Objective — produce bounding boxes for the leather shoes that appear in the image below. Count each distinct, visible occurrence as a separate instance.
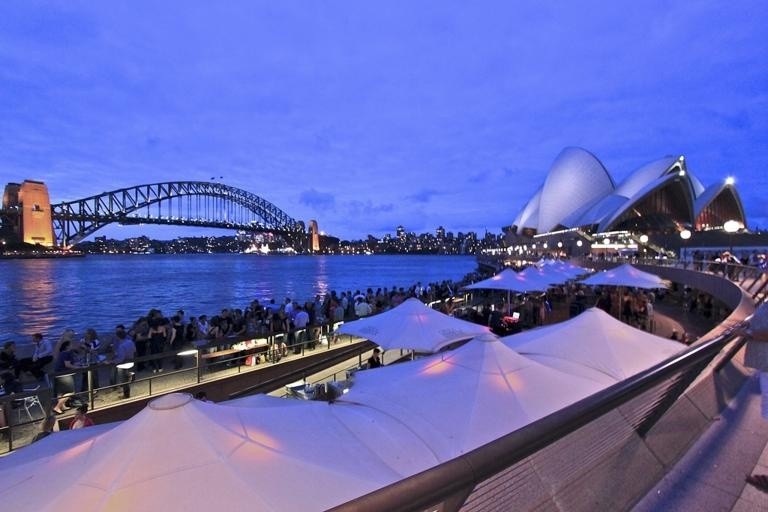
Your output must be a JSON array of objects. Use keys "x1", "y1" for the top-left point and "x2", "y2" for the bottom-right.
[{"x1": 53, "y1": 405, "x2": 71, "y2": 414}]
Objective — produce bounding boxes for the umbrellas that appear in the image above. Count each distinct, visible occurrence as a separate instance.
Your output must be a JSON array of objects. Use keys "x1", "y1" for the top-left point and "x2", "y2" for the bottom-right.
[{"x1": 0, "y1": 391, "x2": 406, "y2": 511}]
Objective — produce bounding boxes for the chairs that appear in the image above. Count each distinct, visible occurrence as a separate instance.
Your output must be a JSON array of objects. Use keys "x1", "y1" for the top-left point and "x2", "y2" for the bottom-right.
[{"x1": 9, "y1": 384, "x2": 47, "y2": 424}]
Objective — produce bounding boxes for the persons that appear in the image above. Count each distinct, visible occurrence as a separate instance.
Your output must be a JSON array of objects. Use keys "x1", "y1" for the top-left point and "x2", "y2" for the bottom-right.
[
  {"x1": 438, "y1": 248, "x2": 767, "y2": 346},
  {"x1": 725, "y1": 302, "x2": 768, "y2": 490},
  {"x1": 0, "y1": 282, "x2": 437, "y2": 443}
]
